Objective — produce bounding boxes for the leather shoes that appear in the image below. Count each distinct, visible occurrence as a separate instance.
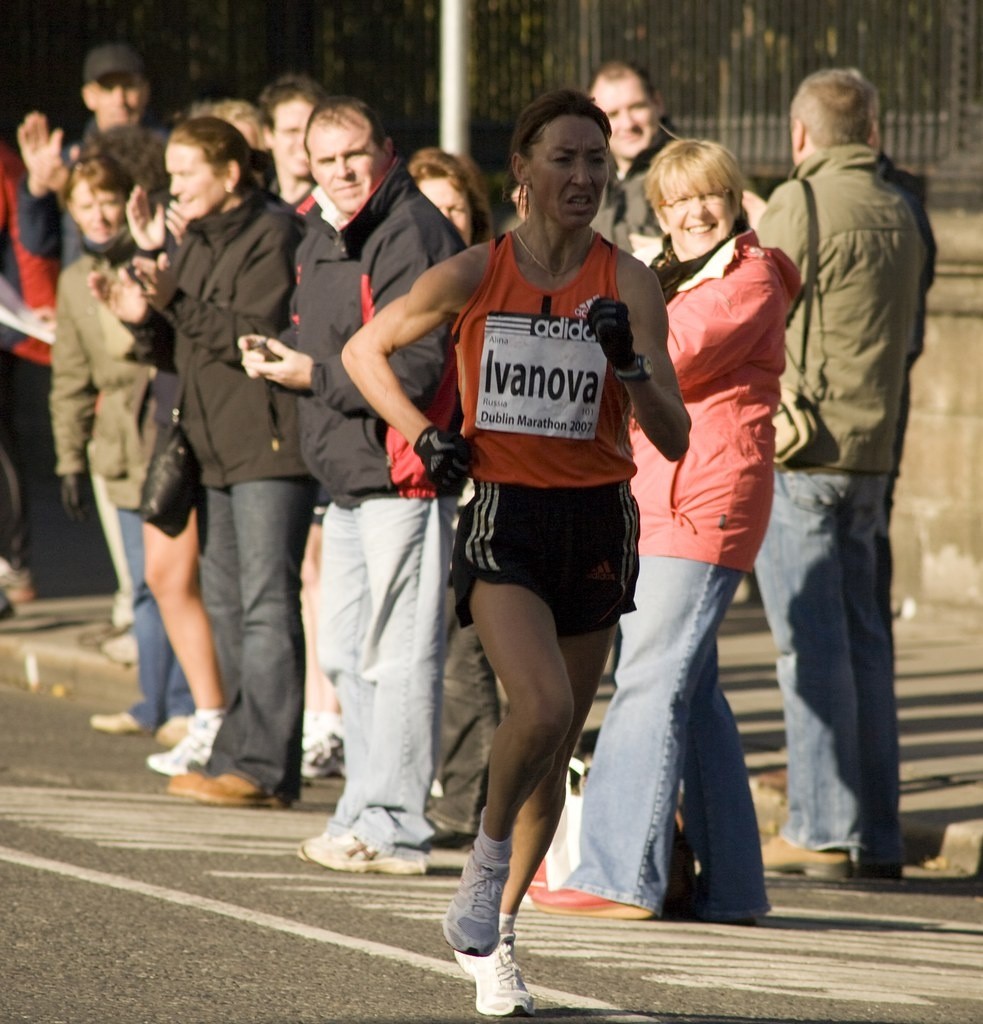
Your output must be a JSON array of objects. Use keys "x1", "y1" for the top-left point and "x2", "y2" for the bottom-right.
[
  {"x1": 532, "y1": 888, "x2": 653, "y2": 919},
  {"x1": 172, "y1": 773, "x2": 292, "y2": 808},
  {"x1": 761, "y1": 835, "x2": 902, "y2": 880}
]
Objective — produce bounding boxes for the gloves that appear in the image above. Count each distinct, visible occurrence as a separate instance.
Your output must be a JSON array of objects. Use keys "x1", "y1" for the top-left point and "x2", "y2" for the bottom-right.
[
  {"x1": 61, "y1": 474, "x2": 97, "y2": 524},
  {"x1": 587, "y1": 298, "x2": 636, "y2": 369},
  {"x1": 412, "y1": 425, "x2": 473, "y2": 496}
]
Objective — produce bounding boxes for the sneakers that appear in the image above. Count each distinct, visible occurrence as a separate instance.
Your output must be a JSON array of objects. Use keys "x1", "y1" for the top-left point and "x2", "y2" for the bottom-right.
[
  {"x1": 298, "y1": 832, "x2": 427, "y2": 875},
  {"x1": 442, "y1": 836, "x2": 510, "y2": 957},
  {"x1": 453, "y1": 931, "x2": 535, "y2": 1016}
]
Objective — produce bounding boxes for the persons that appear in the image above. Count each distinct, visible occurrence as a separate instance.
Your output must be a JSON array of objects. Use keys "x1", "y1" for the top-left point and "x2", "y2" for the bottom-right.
[
  {"x1": 583, "y1": 68, "x2": 709, "y2": 263},
  {"x1": 760, "y1": 68, "x2": 936, "y2": 886},
  {"x1": 521, "y1": 137, "x2": 802, "y2": 921},
  {"x1": 238, "y1": 97, "x2": 495, "y2": 874},
  {"x1": 340, "y1": 89, "x2": 692, "y2": 1019},
  {"x1": 0, "y1": 37, "x2": 487, "y2": 780},
  {"x1": 89, "y1": 111, "x2": 320, "y2": 810}
]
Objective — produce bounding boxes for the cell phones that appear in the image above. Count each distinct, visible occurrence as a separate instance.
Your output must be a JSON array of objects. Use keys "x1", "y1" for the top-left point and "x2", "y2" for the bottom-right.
[{"x1": 249, "y1": 342, "x2": 282, "y2": 363}]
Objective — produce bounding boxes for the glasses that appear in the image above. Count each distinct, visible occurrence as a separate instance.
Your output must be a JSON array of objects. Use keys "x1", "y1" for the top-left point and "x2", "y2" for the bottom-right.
[{"x1": 658, "y1": 189, "x2": 731, "y2": 210}]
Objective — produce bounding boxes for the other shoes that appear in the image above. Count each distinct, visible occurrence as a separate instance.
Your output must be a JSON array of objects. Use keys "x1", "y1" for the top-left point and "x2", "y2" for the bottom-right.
[
  {"x1": 545, "y1": 756, "x2": 589, "y2": 892},
  {"x1": 90, "y1": 713, "x2": 156, "y2": 736},
  {"x1": 299, "y1": 730, "x2": 346, "y2": 778},
  {"x1": 147, "y1": 718, "x2": 220, "y2": 776}
]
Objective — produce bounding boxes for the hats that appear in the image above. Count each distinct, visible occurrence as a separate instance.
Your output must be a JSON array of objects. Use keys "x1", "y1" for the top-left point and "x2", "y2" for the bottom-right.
[{"x1": 83, "y1": 44, "x2": 145, "y2": 81}]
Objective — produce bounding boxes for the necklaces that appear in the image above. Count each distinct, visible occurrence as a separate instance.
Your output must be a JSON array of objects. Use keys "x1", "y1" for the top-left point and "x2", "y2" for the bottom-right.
[{"x1": 515, "y1": 227, "x2": 594, "y2": 275}]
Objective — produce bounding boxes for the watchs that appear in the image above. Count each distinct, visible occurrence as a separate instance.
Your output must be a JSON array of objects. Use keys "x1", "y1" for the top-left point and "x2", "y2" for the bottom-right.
[{"x1": 611, "y1": 353, "x2": 654, "y2": 382}]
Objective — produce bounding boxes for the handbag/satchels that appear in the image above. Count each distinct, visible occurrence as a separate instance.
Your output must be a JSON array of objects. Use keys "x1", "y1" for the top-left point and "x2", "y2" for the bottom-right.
[
  {"x1": 772, "y1": 389, "x2": 817, "y2": 464},
  {"x1": 139, "y1": 426, "x2": 200, "y2": 537}
]
